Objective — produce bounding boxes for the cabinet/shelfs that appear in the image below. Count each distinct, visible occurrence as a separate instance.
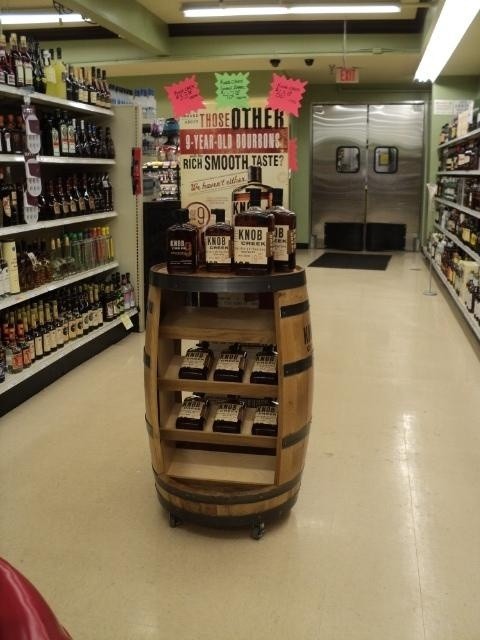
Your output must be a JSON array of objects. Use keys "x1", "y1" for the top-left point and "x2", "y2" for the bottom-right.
[
  {"x1": 1, "y1": 83, "x2": 139, "y2": 392},
  {"x1": 150, "y1": 287, "x2": 282, "y2": 500},
  {"x1": 425, "y1": 137, "x2": 480, "y2": 331}
]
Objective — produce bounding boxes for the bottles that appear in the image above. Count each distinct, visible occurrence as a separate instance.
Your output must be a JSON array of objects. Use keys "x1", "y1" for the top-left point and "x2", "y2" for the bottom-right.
[
  {"x1": 3, "y1": 97, "x2": 116, "y2": 159},
  {"x1": 1, "y1": 33, "x2": 110, "y2": 108},
  {"x1": 57, "y1": 272, "x2": 136, "y2": 322},
  {"x1": 438, "y1": 138, "x2": 480, "y2": 169},
  {"x1": 0, "y1": 300, "x2": 103, "y2": 380},
  {"x1": 434, "y1": 176, "x2": 480, "y2": 211},
  {"x1": 428, "y1": 232, "x2": 480, "y2": 325},
  {"x1": 0, "y1": 164, "x2": 113, "y2": 227},
  {"x1": 176, "y1": 341, "x2": 278, "y2": 436},
  {"x1": 432, "y1": 202, "x2": 480, "y2": 254},
  {"x1": 164, "y1": 164, "x2": 297, "y2": 275},
  {"x1": 0, "y1": 226, "x2": 114, "y2": 295},
  {"x1": 438, "y1": 107, "x2": 480, "y2": 144}
]
